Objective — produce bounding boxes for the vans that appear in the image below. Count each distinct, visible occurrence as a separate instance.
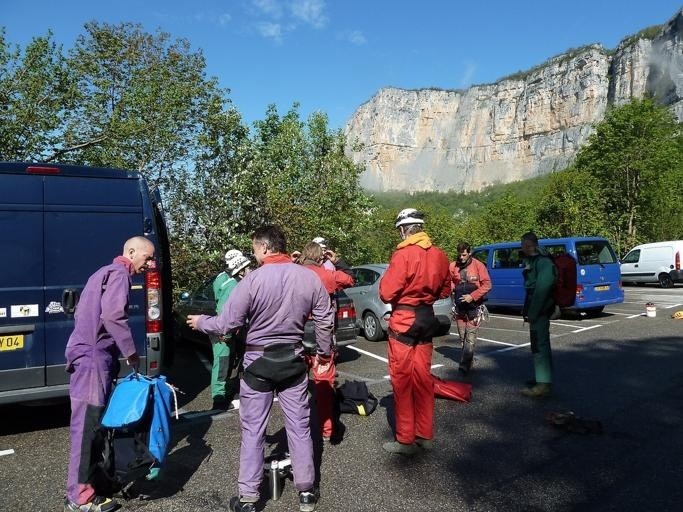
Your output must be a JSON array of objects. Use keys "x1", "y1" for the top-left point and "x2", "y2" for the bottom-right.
[
  {"x1": 618, "y1": 240, "x2": 682, "y2": 288},
  {"x1": 470, "y1": 237, "x2": 624, "y2": 319}
]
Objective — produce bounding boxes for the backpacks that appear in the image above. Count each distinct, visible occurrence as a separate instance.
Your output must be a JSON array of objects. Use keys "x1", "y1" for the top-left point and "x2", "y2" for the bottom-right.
[
  {"x1": 335, "y1": 380, "x2": 378, "y2": 415},
  {"x1": 453, "y1": 283, "x2": 488, "y2": 321},
  {"x1": 535, "y1": 251, "x2": 576, "y2": 309},
  {"x1": 88, "y1": 372, "x2": 179, "y2": 482}
]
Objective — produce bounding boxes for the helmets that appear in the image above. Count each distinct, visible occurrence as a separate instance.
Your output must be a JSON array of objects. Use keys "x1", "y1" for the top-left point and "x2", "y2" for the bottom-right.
[
  {"x1": 227, "y1": 256, "x2": 250, "y2": 276},
  {"x1": 396, "y1": 208, "x2": 424, "y2": 229},
  {"x1": 312, "y1": 236, "x2": 327, "y2": 249},
  {"x1": 225, "y1": 249, "x2": 243, "y2": 262}
]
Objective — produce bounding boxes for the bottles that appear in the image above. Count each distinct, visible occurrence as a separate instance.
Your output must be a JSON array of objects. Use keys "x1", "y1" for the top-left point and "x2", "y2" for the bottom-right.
[{"x1": 268, "y1": 459, "x2": 280, "y2": 500}]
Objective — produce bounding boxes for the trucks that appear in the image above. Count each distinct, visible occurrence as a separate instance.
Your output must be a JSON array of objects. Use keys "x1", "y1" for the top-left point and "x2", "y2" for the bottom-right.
[{"x1": 0, "y1": 161, "x2": 172, "y2": 407}]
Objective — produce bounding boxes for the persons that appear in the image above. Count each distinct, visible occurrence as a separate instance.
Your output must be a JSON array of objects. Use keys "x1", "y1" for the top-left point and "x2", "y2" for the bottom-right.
[
  {"x1": 377, "y1": 206, "x2": 452, "y2": 457},
  {"x1": 517, "y1": 232, "x2": 560, "y2": 399},
  {"x1": 207, "y1": 256, "x2": 253, "y2": 409},
  {"x1": 286, "y1": 241, "x2": 353, "y2": 440},
  {"x1": 310, "y1": 236, "x2": 337, "y2": 271},
  {"x1": 63, "y1": 233, "x2": 154, "y2": 510},
  {"x1": 210, "y1": 248, "x2": 250, "y2": 383},
  {"x1": 183, "y1": 223, "x2": 335, "y2": 511},
  {"x1": 445, "y1": 241, "x2": 492, "y2": 372}
]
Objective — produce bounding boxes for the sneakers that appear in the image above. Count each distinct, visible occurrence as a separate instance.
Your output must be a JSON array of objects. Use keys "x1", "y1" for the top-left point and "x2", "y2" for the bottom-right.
[
  {"x1": 520, "y1": 383, "x2": 551, "y2": 397},
  {"x1": 416, "y1": 438, "x2": 433, "y2": 449},
  {"x1": 298, "y1": 491, "x2": 318, "y2": 512},
  {"x1": 382, "y1": 440, "x2": 416, "y2": 455},
  {"x1": 230, "y1": 496, "x2": 255, "y2": 512},
  {"x1": 62, "y1": 496, "x2": 117, "y2": 512}
]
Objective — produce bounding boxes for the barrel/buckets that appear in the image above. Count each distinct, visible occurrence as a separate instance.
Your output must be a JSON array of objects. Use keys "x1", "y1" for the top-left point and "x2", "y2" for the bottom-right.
[{"x1": 646, "y1": 305, "x2": 657, "y2": 317}]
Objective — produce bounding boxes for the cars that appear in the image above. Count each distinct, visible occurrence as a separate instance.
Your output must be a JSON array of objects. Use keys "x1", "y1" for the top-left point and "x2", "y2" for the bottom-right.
[
  {"x1": 341, "y1": 264, "x2": 453, "y2": 342},
  {"x1": 172, "y1": 264, "x2": 357, "y2": 360}
]
